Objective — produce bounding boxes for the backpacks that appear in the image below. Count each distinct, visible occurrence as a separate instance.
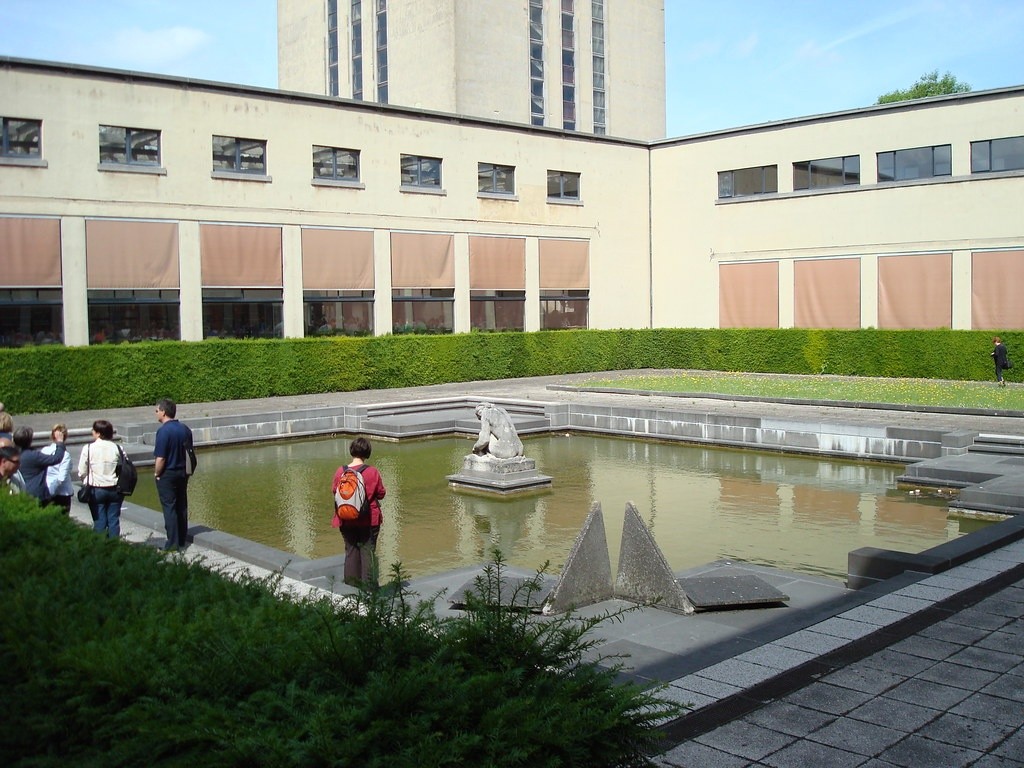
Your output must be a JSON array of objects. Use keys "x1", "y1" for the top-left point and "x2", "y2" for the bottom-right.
[{"x1": 335, "y1": 465, "x2": 381, "y2": 521}]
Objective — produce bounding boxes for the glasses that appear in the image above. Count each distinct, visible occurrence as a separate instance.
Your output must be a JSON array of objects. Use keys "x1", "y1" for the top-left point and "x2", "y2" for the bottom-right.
[
  {"x1": 7, "y1": 459, "x2": 19, "y2": 464},
  {"x1": 156, "y1": 410, "x2": 158, "y2": 412}
]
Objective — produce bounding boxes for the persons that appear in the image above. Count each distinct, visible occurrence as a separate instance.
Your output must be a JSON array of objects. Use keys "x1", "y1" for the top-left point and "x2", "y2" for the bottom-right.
[
  {"x1": 0, "y1": 309, "x2": 449, "y2": 349},
  {"x1": 0, "y1": 402, "x2": 75, "y2": 516},
  {"x1": 472, "y1": 402, "x2": 524, "y2": 459},
  {"x1": 333, "y1": 437, "x2": 386, "y2": 599},
  {"x1": 152, "y1": 399, "x2": 193, "y2": 553},
  {"x1": 78, "y1": 420, "x2": 129, "y2": 539},
  {"x1": 990, "y1": 336, "x2": 1008, "y2": 388}
]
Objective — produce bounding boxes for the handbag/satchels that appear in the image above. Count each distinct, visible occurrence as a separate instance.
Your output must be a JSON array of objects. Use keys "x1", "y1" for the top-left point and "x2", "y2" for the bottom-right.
[
  {"x1": 183, "y1": 440, "x2": 197, "y2": 475},
  {"x1": 115, "y1": 445, "x2": 137, "y2": 496},
  {"x1": 77, "y1": 486, "x2": 91, "y2": 503},
  {"x1": 1002, "y1": 359, "x2": 1012, "y2": 370}
]
